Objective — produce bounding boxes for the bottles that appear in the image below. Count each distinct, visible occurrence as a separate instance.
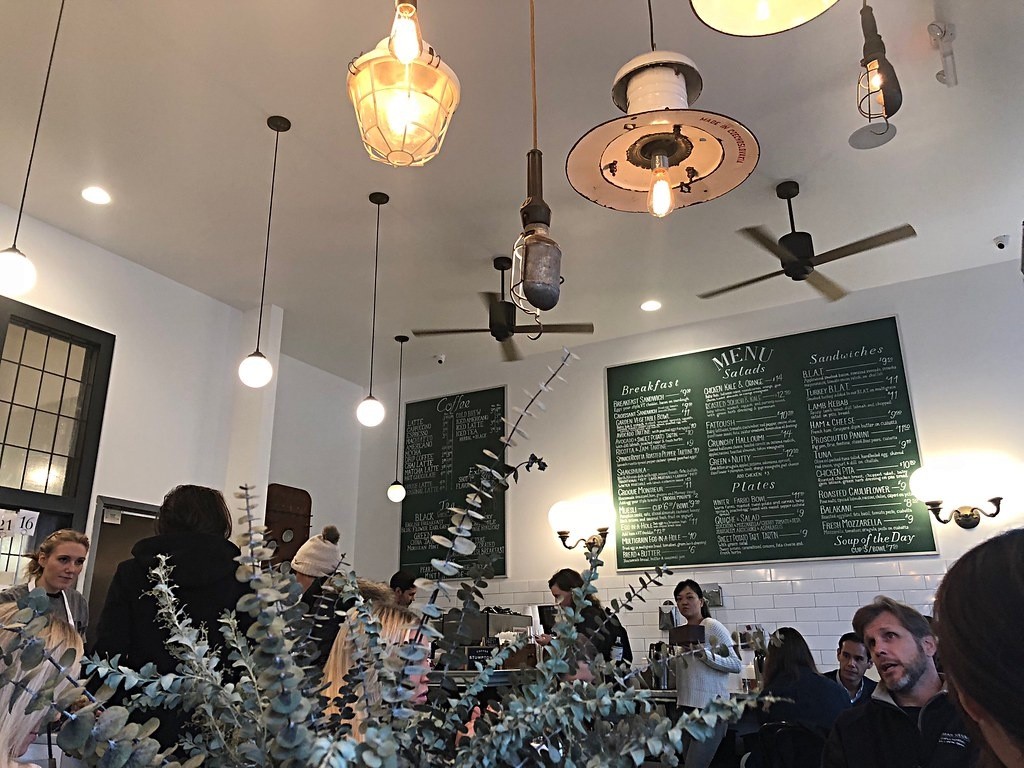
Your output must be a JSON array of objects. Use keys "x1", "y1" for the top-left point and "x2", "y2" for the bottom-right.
[
  {"x1": 610, "y1": 637, "x2": 623, "y2": 663},
  {"x1": 747, "y1": 662, "x2": 755, "y2": 679}
]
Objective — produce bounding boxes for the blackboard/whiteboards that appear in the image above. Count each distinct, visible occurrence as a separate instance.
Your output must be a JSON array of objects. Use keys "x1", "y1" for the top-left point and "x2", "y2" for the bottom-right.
[
  {"x1": 397, "y1": 384, "x2": 509, "y2": 582},
  {"x1": 603, "y1": 313, "x2": 940, "y2": 572}
]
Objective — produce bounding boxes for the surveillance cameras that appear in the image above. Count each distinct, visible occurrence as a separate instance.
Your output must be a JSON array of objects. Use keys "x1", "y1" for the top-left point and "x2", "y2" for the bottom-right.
[
  {"x1": 434, "y1": 354, "x2": 445, "y2": 365},
  {"x1": 993, "y1": 236, "x2": 1009, "y2": 249}
]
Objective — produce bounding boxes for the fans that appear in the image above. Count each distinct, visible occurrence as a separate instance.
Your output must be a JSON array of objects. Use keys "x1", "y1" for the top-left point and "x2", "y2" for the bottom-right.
[
  {"x1": 697, "y1": 181, "x2": 917, "y2": 302},
  {"x1": 412, "y1": 256, "x2": 594, "y2": 362}
]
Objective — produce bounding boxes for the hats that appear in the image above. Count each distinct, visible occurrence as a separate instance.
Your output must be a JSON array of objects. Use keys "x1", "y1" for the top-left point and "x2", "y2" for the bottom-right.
[{"x1": 290, "y1": 524, "x2": 342, "y2": 578}]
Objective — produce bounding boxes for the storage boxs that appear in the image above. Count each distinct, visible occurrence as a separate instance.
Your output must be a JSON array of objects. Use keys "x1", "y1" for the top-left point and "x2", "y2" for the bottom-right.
[{"x1": 433, "y1": 613, "x2": 532, "y2": 647}]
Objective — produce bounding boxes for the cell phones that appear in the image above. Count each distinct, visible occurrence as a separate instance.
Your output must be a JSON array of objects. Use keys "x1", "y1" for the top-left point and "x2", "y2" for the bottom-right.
[{"x1": 533, "y1": 633, "x2": 541, "y2": 639}]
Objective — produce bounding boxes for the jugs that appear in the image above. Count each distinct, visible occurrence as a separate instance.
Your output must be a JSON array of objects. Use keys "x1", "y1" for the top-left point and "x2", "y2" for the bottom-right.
[
  {"x1": 753, "y1": 649, "x2": 767, "y2": 682},
  {"x1": 648, "y1": 641, "x2": 670, "y2": 690}
]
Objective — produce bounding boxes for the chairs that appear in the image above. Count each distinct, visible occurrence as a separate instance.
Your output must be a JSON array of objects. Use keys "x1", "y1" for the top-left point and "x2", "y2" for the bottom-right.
[
  {"x1": 739, "y1": 721, "x2": 823, "y2": 768},
  {"x1": 710, "y1": 728, "x2": 739, "y2": 768}
]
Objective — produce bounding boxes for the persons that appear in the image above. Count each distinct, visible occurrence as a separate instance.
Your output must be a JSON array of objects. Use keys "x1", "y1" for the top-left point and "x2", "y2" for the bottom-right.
[
  {"x1": 933, "y1": 522, "x2": 1024, "y2": 768},
  {"x1": 84, "y1": 484, "x2": 275, "y2": 767},
  {"x1": 535, "y1": 569, "x2": 632, "y2": 731},
  {"x1": 390, "y1": 570, "x2": 416, "y2": 608},
  {"x1": 290, "y1": 523, "x2": 397, "y2": 672},
  {"x1": 821, "y1": 594, "x2": 981, "y2": 768},
  {"x1": 668, "y1": 578, "x2": 742, "y2": 768},
  {"x1": 0, "y1": 528, "x2": 103, "y2": 768},
  {"x1": 744, "y1": 627, "x2": 851, "y2": 768},
  {"x1": 822, "y1": 632, "x2": 879, "y2": 707}
]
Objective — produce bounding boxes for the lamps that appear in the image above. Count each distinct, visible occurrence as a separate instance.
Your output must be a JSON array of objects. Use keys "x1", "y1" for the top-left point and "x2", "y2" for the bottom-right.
[
  {"x1": 855, "y1": 0, "x2": 903, "y2": 136},
  {"x1": 0, "y1": 0, "x2": 65, "y2": 299},
  {"x1": 929, "y1": 21, "x2": 959, "y2": 87},
  {"x1": 239, "y1": 116, "x2": 291, "y2": 389},
  {"x1": 346, "y1": 0, "x2": 461, "y2": 168},
  {"x1": 548, "y1": 499, "x2": 616, "y2": 557},
  {"x1": 356, "y1": 192, "x2": 390, "y2": 426},
  {"x1": 387, "y1": 334, "x2": 410, "y2": 503},
  {"x1": 563, "y1": 0, "x2": 760, "y2": 217},
  {"x1": 509, "y1": 0, "x2": 566, "y2": 340},
  {"x1": 908, "y1": 463, "x2": 1009, "y2": 530},
  {"x1": 688, "y1": 0, "x2": 840, "y2": 38}
]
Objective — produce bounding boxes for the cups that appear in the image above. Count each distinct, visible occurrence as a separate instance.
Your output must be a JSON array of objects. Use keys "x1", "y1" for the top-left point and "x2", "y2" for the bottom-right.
[{"x1": 522, "y1": 604, "x2": 543, "y2": 634}]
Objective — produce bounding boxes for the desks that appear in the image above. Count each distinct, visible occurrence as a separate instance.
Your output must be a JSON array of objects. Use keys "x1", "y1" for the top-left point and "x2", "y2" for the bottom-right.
[
  {"x1": 634, "y1": 689, "x2": 760, "y2": 757},
  {"x1": 427, "y1": 668, "x2": 537, "y2": 687}
]
